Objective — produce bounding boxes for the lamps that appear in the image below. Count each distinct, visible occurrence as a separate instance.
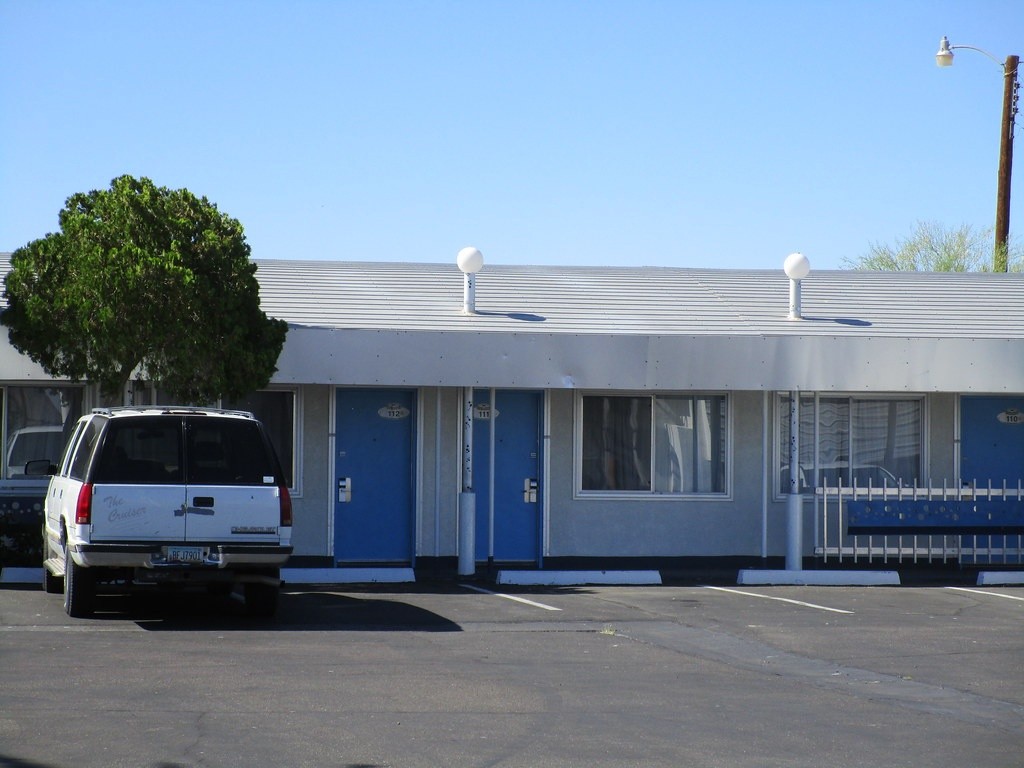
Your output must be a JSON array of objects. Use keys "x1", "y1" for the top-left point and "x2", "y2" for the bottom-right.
[
  {"x1": 784, "y1": 251, "x2": 810, "y2": 320},
  {"x1": 456, "y1": 248, "x2": 484, "y2": 312}
]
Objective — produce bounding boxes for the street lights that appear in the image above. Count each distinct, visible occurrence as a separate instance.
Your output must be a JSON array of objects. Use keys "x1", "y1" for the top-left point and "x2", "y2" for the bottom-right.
[
  {"x1": 781, "y1": 253, "x2": 812, "y2": 572},
  {"x1": 935, "y1": 31, "x2": 1023, "y2": 271},
  {"x1": 457, "y1": 246, "x2": 484, "y2": 579}
]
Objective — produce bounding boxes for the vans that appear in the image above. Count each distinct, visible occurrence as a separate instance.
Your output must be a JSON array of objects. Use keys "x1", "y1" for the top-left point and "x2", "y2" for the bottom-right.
[
  {"x1": 39, "y1": 405, "x2": 295, "y2": 620},
  {"x1": 6, "y1": 422, "x2": 75, "y2": 482}
]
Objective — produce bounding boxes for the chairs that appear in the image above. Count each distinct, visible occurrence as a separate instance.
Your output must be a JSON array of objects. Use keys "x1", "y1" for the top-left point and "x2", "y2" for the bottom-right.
[{"x1": 196, "y1": 441, "x2": 231, "y2": 480}]
